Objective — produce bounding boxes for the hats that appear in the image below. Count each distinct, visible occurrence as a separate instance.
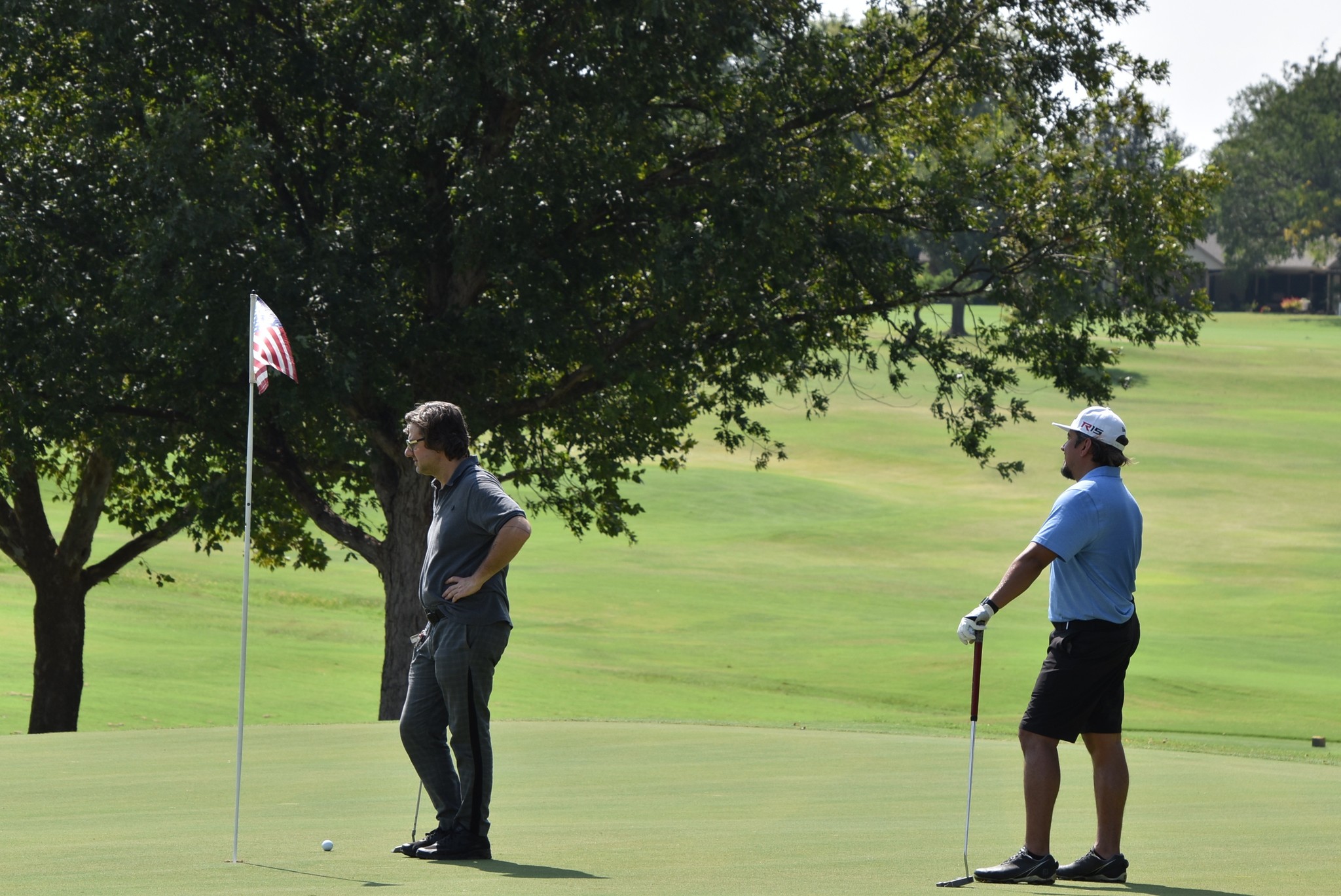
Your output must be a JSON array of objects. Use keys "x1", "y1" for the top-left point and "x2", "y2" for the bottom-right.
[{"x1": 1052, "y1": 406, "x2": 1126, "y2": 451}]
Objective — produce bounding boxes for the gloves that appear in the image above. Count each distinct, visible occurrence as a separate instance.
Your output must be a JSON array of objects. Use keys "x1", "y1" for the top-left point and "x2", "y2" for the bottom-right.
[{"x1": 957, "y1": 604, "x2": 994, "y2": 645}]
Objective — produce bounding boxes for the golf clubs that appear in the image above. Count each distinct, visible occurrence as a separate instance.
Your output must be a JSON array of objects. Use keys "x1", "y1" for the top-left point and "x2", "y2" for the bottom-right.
[
  {"x1": 394, "y1": 780, "x2": 424, "y2": 853},
  {"x1": 936, "y1": 619, "x2": 988, "y2": 888}
]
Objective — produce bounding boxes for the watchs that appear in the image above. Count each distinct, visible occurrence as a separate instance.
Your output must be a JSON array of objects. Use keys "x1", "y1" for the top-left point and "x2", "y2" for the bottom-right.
[{"x1": 980, "y1": 597, "x2": 999, "y2": 615}]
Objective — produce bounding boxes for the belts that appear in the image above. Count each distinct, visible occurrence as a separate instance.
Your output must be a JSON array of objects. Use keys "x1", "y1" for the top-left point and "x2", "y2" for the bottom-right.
[
  {"x1": 1052, "y1": 619, "x2": 1130, "y2": 632},
  {"x1": 425, "y1": 607, "x2": 445, "y2": 624}
]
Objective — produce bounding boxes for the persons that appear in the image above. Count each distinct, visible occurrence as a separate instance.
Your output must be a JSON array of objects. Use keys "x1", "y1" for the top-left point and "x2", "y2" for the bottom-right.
[
  {"x1": 956, "y1": 406, "x2": 1143, "y2": 885},
  {"x1": 399, "y1": 401, "x2": 532, "y2": 859}
]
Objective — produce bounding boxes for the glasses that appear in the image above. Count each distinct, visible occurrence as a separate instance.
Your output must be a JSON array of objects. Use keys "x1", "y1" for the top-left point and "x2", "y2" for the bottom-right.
[{"x1": 406, "y1": 436, "x2": 425, "y2": 452}]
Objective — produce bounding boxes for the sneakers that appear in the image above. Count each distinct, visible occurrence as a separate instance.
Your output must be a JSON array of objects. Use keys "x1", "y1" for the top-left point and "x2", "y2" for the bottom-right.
[
  {"x1": 973, "y1": 846, "x2": 1059, "y2": 886},
  {"x1": 1055, "y1": 846, "x2": 1129, "y2": 884},
  {"x1": 416, "y1": 831, "x2": 492, "y2": 860},
  {"x1": 401, "y1": 826, "x2": 452, "y2": 858}
]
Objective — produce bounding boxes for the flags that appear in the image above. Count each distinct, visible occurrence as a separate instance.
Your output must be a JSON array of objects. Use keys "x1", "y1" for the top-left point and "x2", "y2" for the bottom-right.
[{"x1": 248, "y1": 294, "x2": 298, "y2": 393}]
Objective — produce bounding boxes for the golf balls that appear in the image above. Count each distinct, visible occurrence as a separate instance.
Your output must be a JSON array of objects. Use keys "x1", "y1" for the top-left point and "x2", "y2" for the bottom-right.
[{"x1": 322, "y1": 840, "x2": 333, "y2": 851}]
